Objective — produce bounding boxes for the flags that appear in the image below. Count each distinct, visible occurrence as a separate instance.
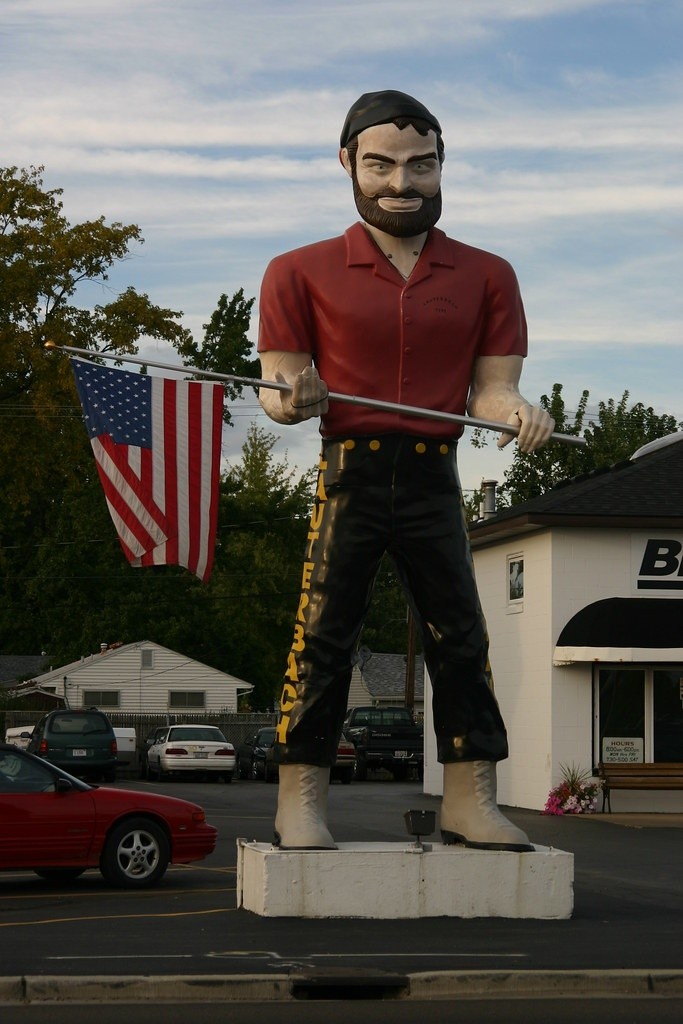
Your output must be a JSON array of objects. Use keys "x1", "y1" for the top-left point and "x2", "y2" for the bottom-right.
[{"x1": 68, "y1": 355, "x2": 224, "y2": 583}]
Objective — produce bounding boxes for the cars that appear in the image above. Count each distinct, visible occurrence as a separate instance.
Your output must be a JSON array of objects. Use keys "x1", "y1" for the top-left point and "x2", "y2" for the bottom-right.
[
  {"x1": 139, "y1": 724, "x2": 236, "y2": 784},
  {"x1": 0, "y1": 742, "x2": 219, "y2": 891},
  {"x1": 263, "y1": 732, "x2": 356, "y2": 786},
  {"x1": 237, "y1": 727, "x2": 277, "y2": 781}
]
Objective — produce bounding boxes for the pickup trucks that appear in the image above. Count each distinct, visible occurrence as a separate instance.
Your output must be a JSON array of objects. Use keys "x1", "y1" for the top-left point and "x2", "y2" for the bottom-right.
[{"x1": 342, "y1": 706, "x2": 423, "y2": 783}]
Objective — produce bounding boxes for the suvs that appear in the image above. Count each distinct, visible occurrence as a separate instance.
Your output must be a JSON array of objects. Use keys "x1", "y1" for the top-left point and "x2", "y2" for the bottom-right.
[{"x1": 27, "y1": 706, "x2": 118, "y2": 783}]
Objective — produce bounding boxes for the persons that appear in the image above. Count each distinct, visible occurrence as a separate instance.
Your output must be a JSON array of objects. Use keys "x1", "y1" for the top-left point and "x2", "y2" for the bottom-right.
[{"x1": 257, "y1": 89, "x2": 558, "y2": 851}]
